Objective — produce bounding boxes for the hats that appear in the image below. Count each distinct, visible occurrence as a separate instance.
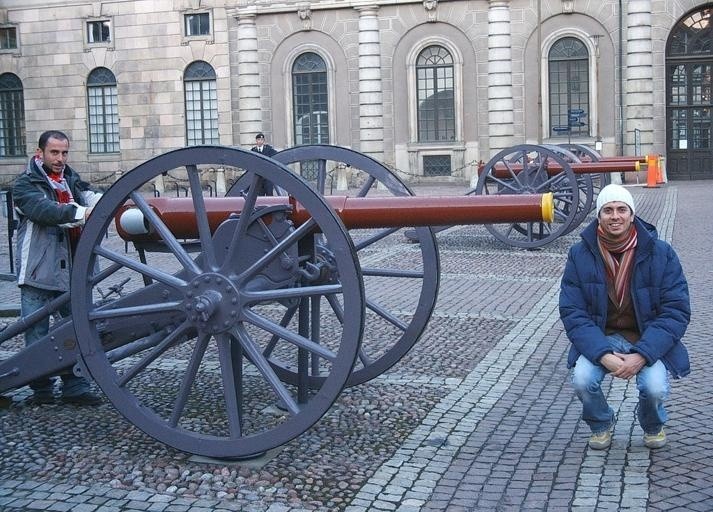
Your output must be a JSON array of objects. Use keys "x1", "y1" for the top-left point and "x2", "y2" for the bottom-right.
[
  {"x1": 595, "y1": 183, "x2": 636, "y2": 219},
  {"x1": 255, "y1": 133, "x2": 264, "y2": 139}
]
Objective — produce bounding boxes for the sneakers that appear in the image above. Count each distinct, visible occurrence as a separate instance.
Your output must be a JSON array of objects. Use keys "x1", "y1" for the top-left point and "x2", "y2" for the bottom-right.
[
  {"x1": 642, "y1": 426, "x2": 667, "y2": 450},
  {"x1": 33, "y1": 390, "x2": 57, "y2": 405},
  {"x1": 587, "y1": 427, "x2": 612, "y2": 451},
  {"x1": 59, "y1": 392, "x2": 103, "y2": 406}
]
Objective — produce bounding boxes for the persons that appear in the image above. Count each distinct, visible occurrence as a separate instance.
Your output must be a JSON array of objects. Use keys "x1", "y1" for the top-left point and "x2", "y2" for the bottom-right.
[
  {"x1": 12, "y1": 131, "x2": 107, "y2": 405},
  {"x1": 559, "y1": 184, "x2": 689, "y2": 449},
  {"x1": 249, "y1": 133, "x2": 281, "y2": 195}
]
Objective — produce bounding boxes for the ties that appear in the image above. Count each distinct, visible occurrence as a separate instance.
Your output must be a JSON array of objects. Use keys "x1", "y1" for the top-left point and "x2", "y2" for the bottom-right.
[{"x1": 258, "y1": 148, "x2": 261, "y2": 153}]
